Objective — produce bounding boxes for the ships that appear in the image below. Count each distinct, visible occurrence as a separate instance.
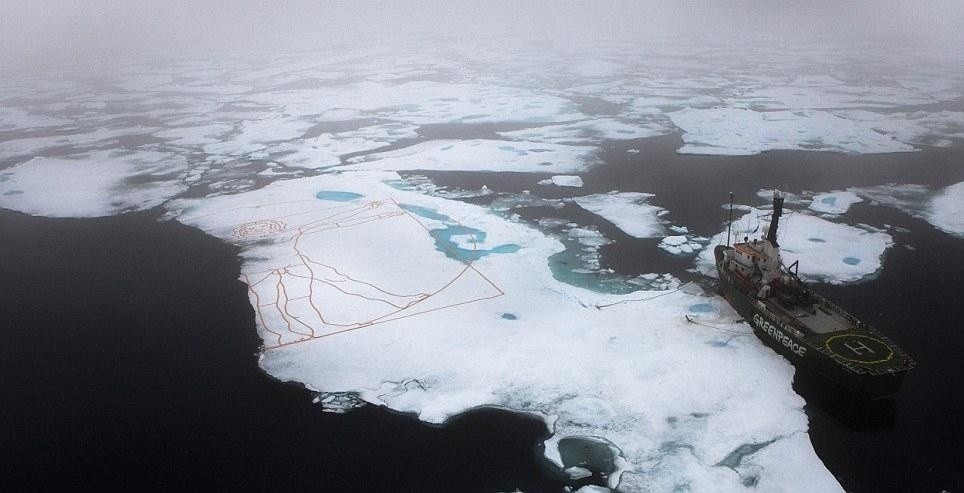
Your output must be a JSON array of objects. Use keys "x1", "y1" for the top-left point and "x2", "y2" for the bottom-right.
[{"x1": 714, "y1": 182, "x2": 913, "y2": 414}]
